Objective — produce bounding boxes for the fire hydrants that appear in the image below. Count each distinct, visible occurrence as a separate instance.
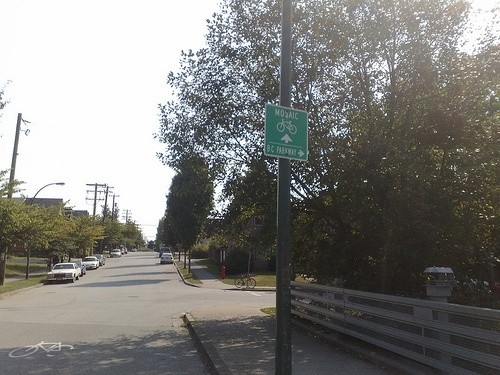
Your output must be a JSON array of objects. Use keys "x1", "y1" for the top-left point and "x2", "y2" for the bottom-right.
[{"x1": 220, "y1": 265, "x2": 226, "y2": 279}]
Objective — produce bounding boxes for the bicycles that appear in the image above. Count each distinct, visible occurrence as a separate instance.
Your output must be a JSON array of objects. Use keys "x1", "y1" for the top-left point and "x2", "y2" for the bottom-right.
[{"x1": 234, "y1": 272, "x2": 257, "y2": 289}]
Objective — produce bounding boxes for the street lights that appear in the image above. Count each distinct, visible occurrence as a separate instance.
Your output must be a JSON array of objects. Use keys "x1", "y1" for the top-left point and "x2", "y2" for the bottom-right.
[{"x1": 25, "y1": 182, "x2": 65, "y2": 279}]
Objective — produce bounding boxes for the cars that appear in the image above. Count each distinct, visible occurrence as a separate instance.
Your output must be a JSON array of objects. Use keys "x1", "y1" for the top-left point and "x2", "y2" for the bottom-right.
[
  {"x1": 80, "y1": 256, "x2": 100, "y2": 270},
  {"x1": 92, "y1": 254, "x2": 105, "y2": 266},
  {"x1": 109, "y1": 247, "x2": 127, "y2": 258},
  {"x1": 160, "y1": 252, "x2": 174, "y2": 264},
  {"x1": 71, "y1": 261, "x2": 86, "y2": 277},
  {"x1": 47, "y1": 262, "x2": 82, "y2": 284}
]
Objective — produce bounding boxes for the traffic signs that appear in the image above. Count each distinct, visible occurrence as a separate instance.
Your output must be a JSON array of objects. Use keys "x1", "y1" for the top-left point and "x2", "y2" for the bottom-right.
[{"x1": 264, "y1": 103, "x2": 309, "y2": 162}]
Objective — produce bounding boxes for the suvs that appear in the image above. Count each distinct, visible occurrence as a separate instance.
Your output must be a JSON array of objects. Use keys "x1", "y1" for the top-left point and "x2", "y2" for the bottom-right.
[{"x1": 159, "y1": 247, "x2": 172, "y2": 258}]
